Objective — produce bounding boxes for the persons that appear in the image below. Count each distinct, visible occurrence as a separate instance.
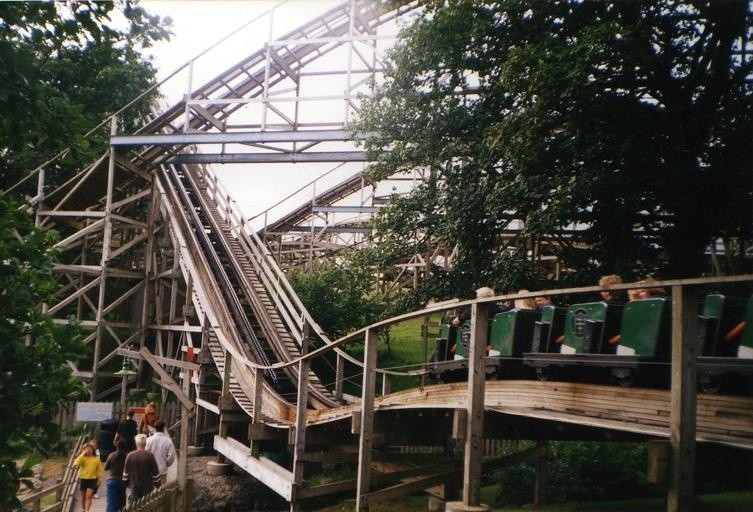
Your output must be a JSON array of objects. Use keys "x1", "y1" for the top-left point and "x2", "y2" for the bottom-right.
[
  {"x1": 450, "y1": 275, "x2": 667, "y2": 334},
  {"x1": 71, "y1": 400, "x2": 176, "y2": 512}
]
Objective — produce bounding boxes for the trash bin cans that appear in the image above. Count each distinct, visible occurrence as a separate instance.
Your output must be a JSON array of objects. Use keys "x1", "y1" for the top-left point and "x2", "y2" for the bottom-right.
[{"x1": 100, "y1": 419, "x2": 119, "y2": 462}]
